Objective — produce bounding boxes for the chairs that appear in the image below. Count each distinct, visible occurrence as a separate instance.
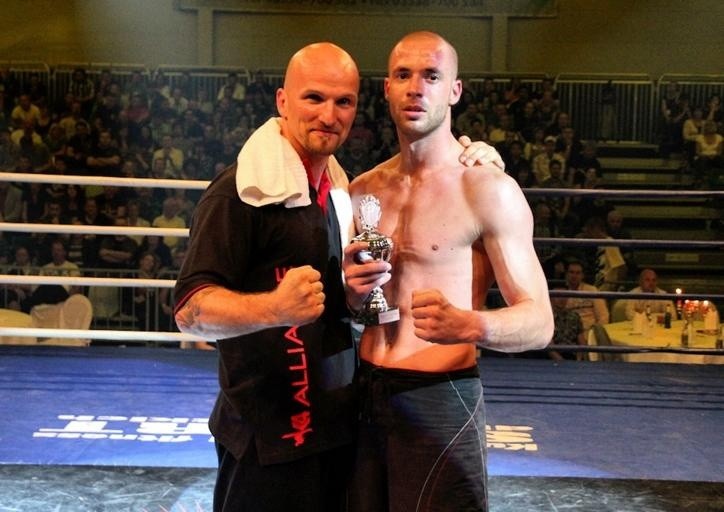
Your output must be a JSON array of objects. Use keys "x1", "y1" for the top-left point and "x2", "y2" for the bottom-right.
[
  {"x1": 40, "y1": 280, "x2": 122, "y2": 346},
  {"x1": 612, "y1": 299, "x2": 627, "y2": 323}
]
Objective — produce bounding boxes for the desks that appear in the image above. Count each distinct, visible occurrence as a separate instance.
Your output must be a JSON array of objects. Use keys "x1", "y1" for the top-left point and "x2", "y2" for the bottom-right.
[{"x1": 0, "y1": 309, "x2": 35, "y2": 345}]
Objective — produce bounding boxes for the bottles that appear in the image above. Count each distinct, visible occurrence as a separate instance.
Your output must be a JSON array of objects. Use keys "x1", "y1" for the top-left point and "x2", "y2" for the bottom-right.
[
  {"x1": 663, "y1": 304, "x2": 672, "y2": 329},
  {"x1": 675, "y1": 300, "x2": 682, "y2": 320},
  {"x1": 644, "y1": 305, "x2": 653, "y2": 323}
]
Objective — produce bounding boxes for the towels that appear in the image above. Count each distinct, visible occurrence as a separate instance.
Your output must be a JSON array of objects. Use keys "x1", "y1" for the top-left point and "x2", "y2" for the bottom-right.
[{"x1": 235, "y1": 116, "x2": 356, "y2": 290}]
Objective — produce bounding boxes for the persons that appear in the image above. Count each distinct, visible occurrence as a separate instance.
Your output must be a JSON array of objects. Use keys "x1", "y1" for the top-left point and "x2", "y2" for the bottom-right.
[
  {"x1": 172, "y1": 41, "x2": 509, "y2": 511},
  {"x1": 348, "y1": 29, "x2": 554, "y2": 511}
]
